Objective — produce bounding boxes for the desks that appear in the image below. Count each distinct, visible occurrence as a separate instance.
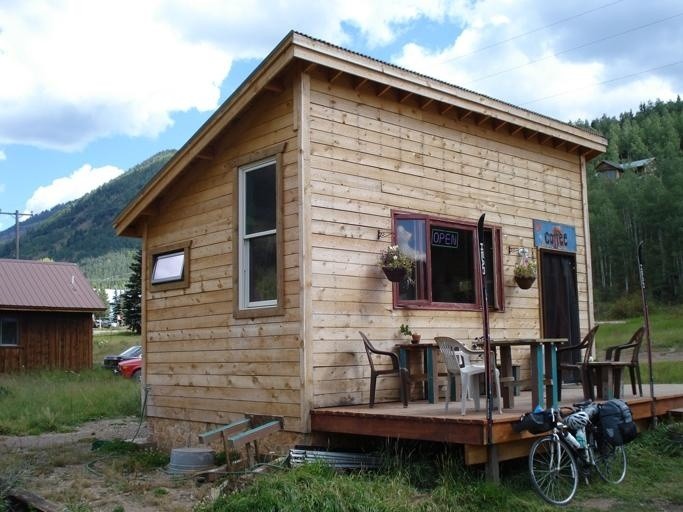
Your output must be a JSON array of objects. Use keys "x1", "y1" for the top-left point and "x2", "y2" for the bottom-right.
[
  {"x1": 473, "y1": 337, "x2": 568, "y2": 412},
  {"x1": 395, "y1": 342, "x2": 497, "y2": 403}
]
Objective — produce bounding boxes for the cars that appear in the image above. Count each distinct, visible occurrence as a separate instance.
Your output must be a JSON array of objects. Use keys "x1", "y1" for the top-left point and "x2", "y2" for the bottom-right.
[
  {"x1": 111, "y1": 355, "x2": 144, "y2": 383},
  {"x1": 102, "y1": 346, "x2": 141, "y2": 371},
  {"x1": 93, "y1": 315, "x2": 119, "y2": 331}
]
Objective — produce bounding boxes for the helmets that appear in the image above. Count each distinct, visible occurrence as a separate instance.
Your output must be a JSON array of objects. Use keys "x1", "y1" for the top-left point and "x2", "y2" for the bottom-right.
[{"x1": 562, "y1": 410, "x2": 592, "y2": 431}]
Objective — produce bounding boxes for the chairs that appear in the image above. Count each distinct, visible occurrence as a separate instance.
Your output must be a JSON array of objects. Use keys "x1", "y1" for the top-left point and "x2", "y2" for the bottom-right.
[
  {"x1": 357, "y1": 329, "x2": 407, "y2": 409},
  {"x1": 587, "y1": 326, "x2": 645, "y2": 399},
  {"x1": 557, "y1": 324, "x2": 599, "y2": 401},
  {"x1": 434, "y1": 336, "x2": 503, "y2": 416}
]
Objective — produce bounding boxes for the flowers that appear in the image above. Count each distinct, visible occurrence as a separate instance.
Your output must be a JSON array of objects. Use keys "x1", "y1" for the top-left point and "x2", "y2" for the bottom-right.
[
  {"x1": 513, "y1": 258, "x2": 536, "y2": 279},
  {"x1": 377, "y1": 244, "x2": 414, "y2": 292}
]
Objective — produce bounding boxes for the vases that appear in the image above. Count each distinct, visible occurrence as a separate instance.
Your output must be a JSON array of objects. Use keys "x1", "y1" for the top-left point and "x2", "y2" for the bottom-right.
[
  {"x1": 381, "y1": 268, "x2": 409, "y2": 283},
  {"x1": 513, "y1": 275, "x2": 536, "y2": 289}
]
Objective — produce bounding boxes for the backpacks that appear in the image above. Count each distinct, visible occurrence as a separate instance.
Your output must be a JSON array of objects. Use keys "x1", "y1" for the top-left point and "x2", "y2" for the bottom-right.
[{"x1": 596, "y1": 399, "x2": 638, "y2": 447}]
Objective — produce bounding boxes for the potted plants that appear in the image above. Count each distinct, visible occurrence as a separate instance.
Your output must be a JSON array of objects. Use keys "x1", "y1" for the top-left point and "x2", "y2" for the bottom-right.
[{"x1": 400, "y1": 323, "x2": 421, "y2": 344}]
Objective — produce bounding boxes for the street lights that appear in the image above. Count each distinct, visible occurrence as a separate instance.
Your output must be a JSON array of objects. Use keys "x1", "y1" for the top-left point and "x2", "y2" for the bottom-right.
[{"x1": 595, "y1": 154, "x2": 658, "y2": 428}]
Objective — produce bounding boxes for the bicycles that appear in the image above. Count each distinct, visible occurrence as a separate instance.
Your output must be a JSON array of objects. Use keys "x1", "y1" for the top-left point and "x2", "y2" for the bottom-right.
[{"x1": 511, "y1": 399, "x2": 629, "y2": 503}]
